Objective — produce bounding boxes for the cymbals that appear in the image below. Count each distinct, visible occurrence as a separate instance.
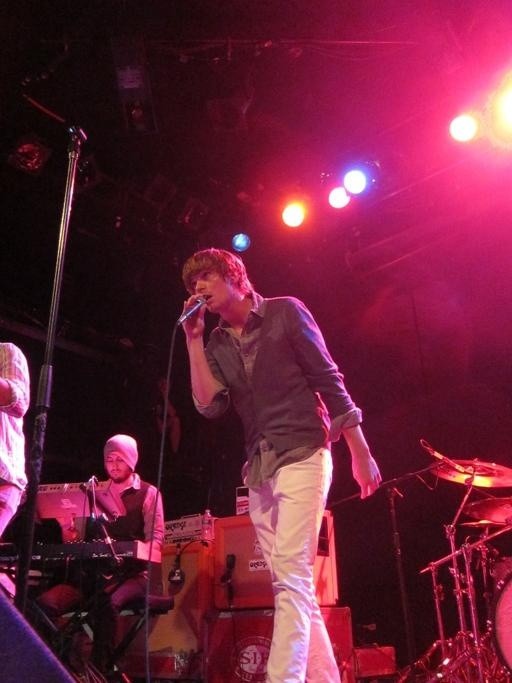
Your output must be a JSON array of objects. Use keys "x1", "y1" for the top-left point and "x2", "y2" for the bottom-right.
[
  {"x1": 429, "y1": 459, "x2": 512, "y2": 487},
  {"x1": 463, "y1": 498, "x2": 511, "y2": 525}
]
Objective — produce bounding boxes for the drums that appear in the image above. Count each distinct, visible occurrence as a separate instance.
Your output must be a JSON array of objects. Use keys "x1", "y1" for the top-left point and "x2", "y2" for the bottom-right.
[{"x1": 492, "y1": 572, "x2": 511, "y2": 672}]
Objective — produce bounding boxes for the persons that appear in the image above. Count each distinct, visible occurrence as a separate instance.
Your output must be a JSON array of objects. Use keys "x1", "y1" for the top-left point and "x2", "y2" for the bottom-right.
[
  {"x1": 179, "y1": 246, "x2": 384, "y2": 681},
  {"x1": 1, "y1": 339, "x2": 34, "y2": 545},
  {"x1": 26, "y1": 432, "x2": 165, "y2": 681}
]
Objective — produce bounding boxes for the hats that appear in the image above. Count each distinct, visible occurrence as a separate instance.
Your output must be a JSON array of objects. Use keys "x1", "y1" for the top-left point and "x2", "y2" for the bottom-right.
[{"x1": 102, "y1": 432, "x2": 140, "y2": 472}]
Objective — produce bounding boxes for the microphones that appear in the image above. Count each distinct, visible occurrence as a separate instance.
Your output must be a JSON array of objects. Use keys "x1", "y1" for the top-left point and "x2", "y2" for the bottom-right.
[{"x1": 177, "y1": 297, "x2": 206, "y2": 325}]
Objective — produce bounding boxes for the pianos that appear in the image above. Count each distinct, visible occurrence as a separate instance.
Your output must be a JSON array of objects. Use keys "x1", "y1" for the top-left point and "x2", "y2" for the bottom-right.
[{"x1": 0, "y1": 540, "x2": 162, "y2": 567}]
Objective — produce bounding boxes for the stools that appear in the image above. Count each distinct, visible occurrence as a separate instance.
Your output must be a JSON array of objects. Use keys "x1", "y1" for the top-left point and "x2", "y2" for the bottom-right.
[{"x1": 87, "y1": 592, "x2": 174, "y2": 683}]
